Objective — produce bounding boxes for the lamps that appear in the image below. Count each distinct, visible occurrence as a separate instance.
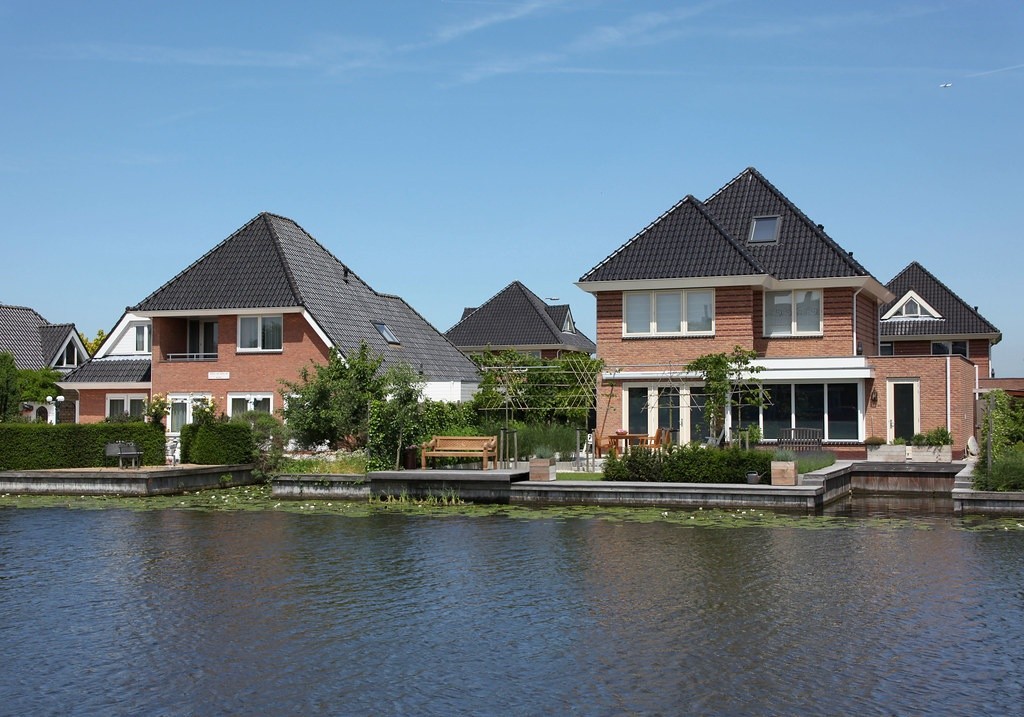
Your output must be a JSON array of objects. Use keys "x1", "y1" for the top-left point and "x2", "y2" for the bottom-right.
[{"x1": 872, "y1": 387, "x2": 878, "y2": 402}]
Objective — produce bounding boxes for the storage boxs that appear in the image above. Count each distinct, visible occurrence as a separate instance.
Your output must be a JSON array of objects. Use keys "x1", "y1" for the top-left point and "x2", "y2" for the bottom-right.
[{"x1": 770, "y1": 450, "x2": 798, "y2": 485}]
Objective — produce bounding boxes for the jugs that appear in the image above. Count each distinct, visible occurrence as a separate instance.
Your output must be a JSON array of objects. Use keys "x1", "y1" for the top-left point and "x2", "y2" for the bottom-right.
[{"x1": 745, "y1": 470, "x2": 767, "y2": 485}]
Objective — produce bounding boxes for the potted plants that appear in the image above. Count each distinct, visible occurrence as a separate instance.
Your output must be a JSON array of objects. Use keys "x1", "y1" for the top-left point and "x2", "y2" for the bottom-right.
[
  {"x1": 530, "y1": 446, "x2": 556, "y2": 482},
  {"x1": 865, "y1": 426, "x2": 954, "y2": 463}
]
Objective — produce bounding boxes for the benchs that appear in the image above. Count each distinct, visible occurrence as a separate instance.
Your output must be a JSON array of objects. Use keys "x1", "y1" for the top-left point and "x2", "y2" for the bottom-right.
[
  {"x1": 777, "y1": 428, "x2": 823, "y2": 451},
  {"x1": 421, "y1": 435, "x2": 498, "y2": 470},
  {"x1": 105, "y1": 443, "x2": 144, "y2": 469}
]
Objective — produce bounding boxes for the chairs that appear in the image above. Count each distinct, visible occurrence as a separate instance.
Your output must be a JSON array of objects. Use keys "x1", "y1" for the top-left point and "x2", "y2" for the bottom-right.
[
  {"x1": 638, "y1": 429, "x2": 670, "y2": 452},
  {"x1": 593, "y1": 429, "x2": 612, "y2": 458}
]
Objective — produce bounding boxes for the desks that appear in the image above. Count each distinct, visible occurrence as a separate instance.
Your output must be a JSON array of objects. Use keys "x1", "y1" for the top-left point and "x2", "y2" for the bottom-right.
[{"x1": 608, "y1": 433, "x2": 649, "y2": 453}]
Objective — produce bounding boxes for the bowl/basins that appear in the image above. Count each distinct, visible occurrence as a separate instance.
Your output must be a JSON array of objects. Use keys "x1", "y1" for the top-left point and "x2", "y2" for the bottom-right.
[{"x1": 616, "y1": 431, "x2": 628, "y2": 435}]
